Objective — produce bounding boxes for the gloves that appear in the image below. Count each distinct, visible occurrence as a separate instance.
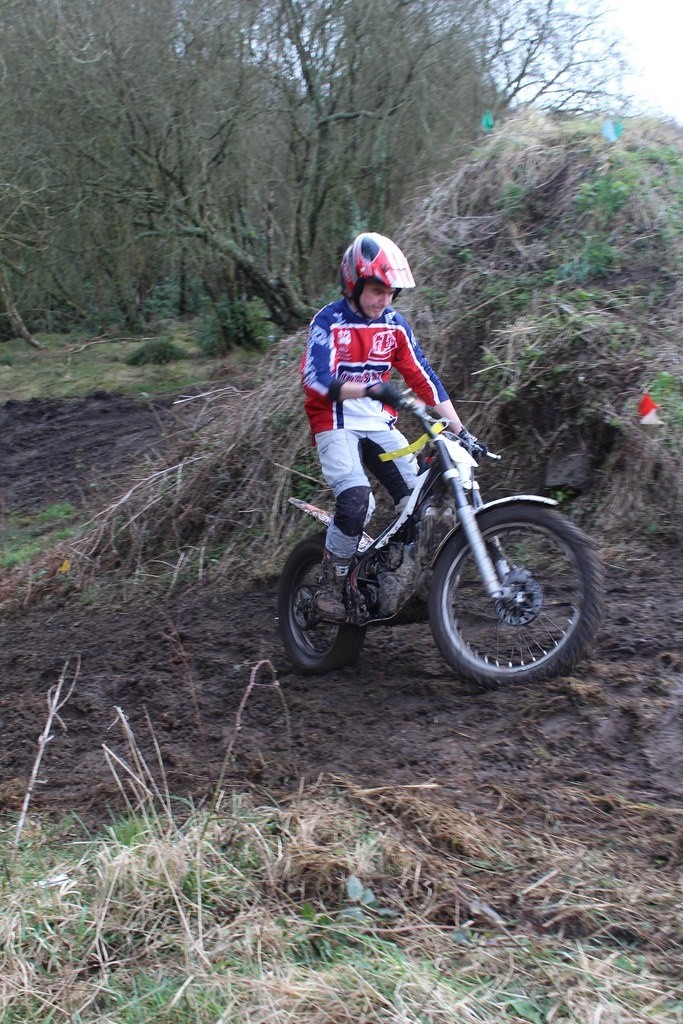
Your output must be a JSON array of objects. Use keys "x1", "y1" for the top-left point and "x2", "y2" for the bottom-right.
[
  {"x1": 365, "y1": 380, "x2": 401, "y2": 409},
  {"x1": 457, "y1": 429, "x2": 488, "y2": 457}
]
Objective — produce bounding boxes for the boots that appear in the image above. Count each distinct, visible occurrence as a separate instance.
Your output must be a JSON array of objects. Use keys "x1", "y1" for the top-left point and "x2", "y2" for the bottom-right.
[{"x1": 313, "y1": 551, "x2": 353, "y2": 621}]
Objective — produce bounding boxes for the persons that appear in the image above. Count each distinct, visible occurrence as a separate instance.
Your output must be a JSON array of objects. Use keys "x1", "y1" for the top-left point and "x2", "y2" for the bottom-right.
[{"x1": 298, "y1": 228, "x2": 490, "y2": 627}]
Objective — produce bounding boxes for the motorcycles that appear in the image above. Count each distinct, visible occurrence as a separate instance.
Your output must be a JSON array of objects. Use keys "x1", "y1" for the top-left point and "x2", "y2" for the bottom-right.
[{"x1": 276, "y1": 387, "x2": 604, "y2": 689}]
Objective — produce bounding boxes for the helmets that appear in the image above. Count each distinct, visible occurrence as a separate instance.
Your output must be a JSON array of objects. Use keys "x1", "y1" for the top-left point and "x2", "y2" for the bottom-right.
[{"x1": 340, "y1": 233, "x2": 416, "y2": 297}]
